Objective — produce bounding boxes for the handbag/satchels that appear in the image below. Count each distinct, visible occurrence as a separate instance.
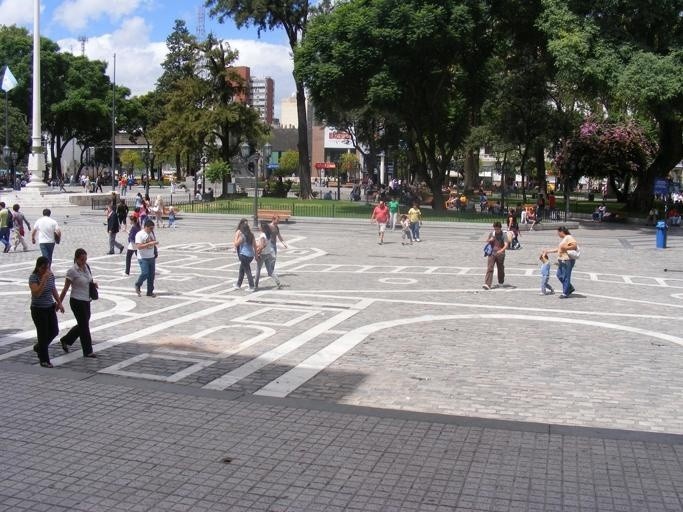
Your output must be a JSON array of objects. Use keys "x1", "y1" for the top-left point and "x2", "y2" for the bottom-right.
[
  {"x1": 6, "y1": 209, "x2": 12, "y2": 228},
  {"x1": 86, "y1": 263, "x2": 98, "y2": 300},
  {"x1": 152, "y1": 231, "x2": 158, "y2": 257},
  {"x1": 482, "y1": 231, "x2": 494, "y2": 256},
  {"x1": 566, "y1": 243, "x2": 580, "y2": 259}
]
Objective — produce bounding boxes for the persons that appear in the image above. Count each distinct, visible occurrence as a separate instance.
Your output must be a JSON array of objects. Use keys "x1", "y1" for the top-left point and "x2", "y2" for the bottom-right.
[
  {"x1": 0, "y1": 201, "x2": 32, "y2": 253},
  {"x1": 132, "y1": 219, "x2": 159, "y2": 301},
  {"x1": 229, "y1": 221, "x2": 255, "y2": 295},
  {"x1": 107, "y1": 174, "x2": 177, "y2": 255},
  {"x1": 50, "y1": 173, "x2": 103, "y2": 193},
  {"x1": 480, "y1": 221, "x2": 508, "y2": 291},
  {"x1": 315, "y1": 175, "x2": 361, "y2": 202},
  {"x1": 234, "y1": 179, "x2": 270, "y2": 194},
  {"x1": 538, "y1": 253, "x2": 556, "y2": 296},
  {"x1": 250, "y1": 222, "x2": 284, "y2": 291},
  {"x1": 122, "y1": 215, "x2": 143, "y2": 278},
  {"x1": 15, "y1": 172, "x2": 31, "y2": 190},
  {"x1": 363, "y1": 176, "x2": 426, "y2": 245},
  {"x1": 579, "y1": 182, "x2": 612, "y2": 222},
  {"x1": 646, "y1": 199, "x2": 683, "y2": 226},
  {"x1": 27, "y1": 256, "x2": 65, "y2": 370},
  {"x1": 196, "y1": 176, "x2": 214, "y2": 201},
  {"x1": 442, "y1": 181, "x2": 561, "y2": 231},
  {"x1": 30, "y1": 207, "x2": 63, "y2": 273},
  {"x1": 54, "y1": 246, "x2": 98, "y2": 362},
  {"x1": 545, "y1": 224, "x2": 582, "y2": 300}
]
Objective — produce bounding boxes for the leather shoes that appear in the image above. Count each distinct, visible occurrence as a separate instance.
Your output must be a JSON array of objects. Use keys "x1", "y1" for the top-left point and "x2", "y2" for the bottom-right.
[
  {"x1": 41, "y1": 361, "x2": 53, "y2": 368},
  {"x1": 60, "y1": 339, "x2": 68, "y2": 352},
  {"x1": 84, "y1": 352, "x2": 97, "y2": 358},
  {"x1": 34, "y1": 343, "x2": 40, "y2": 358}
]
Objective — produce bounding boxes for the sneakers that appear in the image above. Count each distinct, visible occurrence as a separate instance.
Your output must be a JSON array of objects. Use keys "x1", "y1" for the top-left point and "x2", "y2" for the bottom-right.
[
  {"x1": 232, "y1": 283, "x2": 239, "y2": 289},
  {"x1": 244, "y1": 288, "x2": 254, "y2": 291}
]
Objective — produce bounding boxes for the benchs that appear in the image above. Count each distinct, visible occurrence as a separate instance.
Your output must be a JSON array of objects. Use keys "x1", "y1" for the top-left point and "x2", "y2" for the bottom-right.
[
  {"x1": 147, "y1": 208, "x2": 177, "y2": 220},
  {"x1": 257, "y1": 210, "x2": 291, "y2": 223}
]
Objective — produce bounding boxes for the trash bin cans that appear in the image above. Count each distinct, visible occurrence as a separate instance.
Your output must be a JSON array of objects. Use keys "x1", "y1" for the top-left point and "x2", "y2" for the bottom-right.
[{"x1": 655, "y1": 220, "x2": 669, "y2": 248}]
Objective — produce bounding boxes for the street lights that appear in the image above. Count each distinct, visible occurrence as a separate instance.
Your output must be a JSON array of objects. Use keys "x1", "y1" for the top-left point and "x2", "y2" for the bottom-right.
[
  {"x1": 2, "y1": 142, "x2": 12, "y2": 191},
  {"x1": 239, "y1": 139, "x2": 273, "y2": 234},
  {"x1": 495, "y1": 158, "x2": 509, "y2": 193}
]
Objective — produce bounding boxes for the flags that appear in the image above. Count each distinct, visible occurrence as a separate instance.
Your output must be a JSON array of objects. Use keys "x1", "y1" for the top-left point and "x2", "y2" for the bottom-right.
[{"x1": 0, "y1": 66, "x2": 19, "y2": 95}]
